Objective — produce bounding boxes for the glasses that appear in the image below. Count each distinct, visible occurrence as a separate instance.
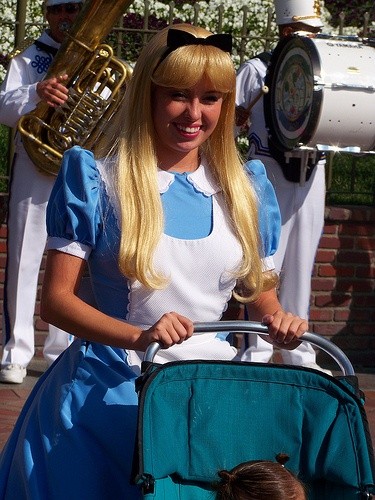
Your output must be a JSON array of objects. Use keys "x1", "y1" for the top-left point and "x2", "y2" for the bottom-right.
[{"x1": 48, "y1": 4, "x2": 81, "y2": 14}]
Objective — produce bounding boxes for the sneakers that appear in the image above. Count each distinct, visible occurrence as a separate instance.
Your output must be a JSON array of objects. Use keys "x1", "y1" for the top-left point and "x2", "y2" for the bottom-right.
[{"x1": 0, "y1": 364, "x2": 26, "y2": 383}]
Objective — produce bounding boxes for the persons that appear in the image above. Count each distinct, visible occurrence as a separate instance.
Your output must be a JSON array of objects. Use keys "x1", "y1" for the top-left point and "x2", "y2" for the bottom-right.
[
  {"x1": 215, "y1": 453, "x2": 309, "y2": 500},
  {"x1": 233, "y1": 1, "x2": 336, "y2": 380},
  {"x1": 2, "y1": 1, "x2": 117, "y2": 384},
  {"x1": 0, "y1": 24, "x2": 309, "y2": 500}
]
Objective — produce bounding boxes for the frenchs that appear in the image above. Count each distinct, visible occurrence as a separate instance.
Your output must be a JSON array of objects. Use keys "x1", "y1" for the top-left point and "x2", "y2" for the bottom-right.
[{"x1": 17, "y1": 0, "x2": 134, "y2": 176}]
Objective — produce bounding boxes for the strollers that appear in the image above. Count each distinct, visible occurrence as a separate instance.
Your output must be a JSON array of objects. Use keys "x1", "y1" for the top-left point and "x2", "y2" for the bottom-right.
[{"x1": 137, "y1": 320, "x2": 375, "y2": 500}]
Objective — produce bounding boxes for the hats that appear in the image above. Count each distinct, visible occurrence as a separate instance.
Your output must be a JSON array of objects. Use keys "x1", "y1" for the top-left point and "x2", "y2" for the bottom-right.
[
  {"x1": 275, "y1": 0, "x2": 324, "y2": 27},
  {"x1": 45, "y1": 0, "x2": 81, "y2": 7}
]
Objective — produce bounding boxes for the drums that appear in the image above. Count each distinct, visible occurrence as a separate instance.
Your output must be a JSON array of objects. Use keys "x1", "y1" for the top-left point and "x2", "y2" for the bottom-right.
[{"x1": 267, "y1": 32, "x2": 375, "y2": 157}]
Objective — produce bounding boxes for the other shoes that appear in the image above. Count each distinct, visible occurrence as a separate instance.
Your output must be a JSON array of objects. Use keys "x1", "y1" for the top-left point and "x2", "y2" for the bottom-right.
[{"x1": 313, "y1": 365, "x2": 332, "y2": 376}]
